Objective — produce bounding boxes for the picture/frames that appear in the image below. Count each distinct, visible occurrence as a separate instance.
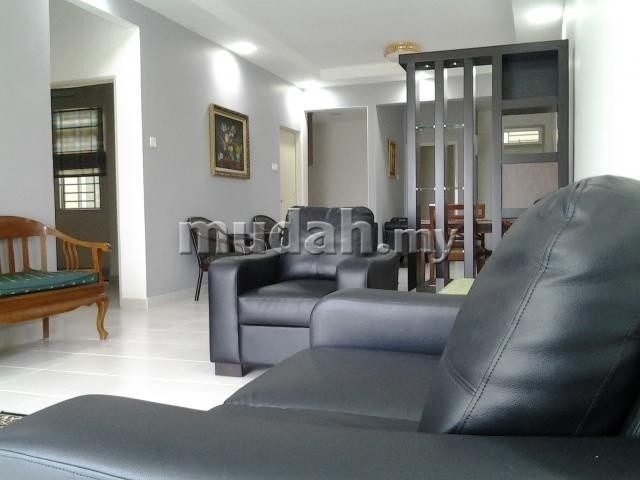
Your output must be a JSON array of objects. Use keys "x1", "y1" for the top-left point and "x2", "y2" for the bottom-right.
[
  {"x1": 208, "y1": 103, "x2": 252, "y2": 180},
  {"x1": 387, "y1": 139, "x2": 399, "y2": 181}
]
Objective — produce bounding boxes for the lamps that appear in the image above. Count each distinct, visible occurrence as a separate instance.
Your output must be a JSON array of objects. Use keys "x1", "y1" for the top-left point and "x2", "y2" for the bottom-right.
[{"x1": 383, "y1": 40, "x2": 423, "y2": 63}]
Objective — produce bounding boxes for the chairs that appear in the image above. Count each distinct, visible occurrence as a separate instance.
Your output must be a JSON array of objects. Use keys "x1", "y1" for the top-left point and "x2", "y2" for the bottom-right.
[
  {"x1": 208, "y1": 205, "x2": 401, "y2": 378},
  {"x1": 382, "y1": 201, "x2": 524, "y2": 285},
  {"x1": 186, "y1": 213, "x2": 268, "y2": 302},
  {"x1": 1, "y1": 215, "x2": 113, "y2": 343},
  {"x1": 0, "y1": 175, "x2": 640, "y2": 480},
  {"x1": 249, "y1": 212, "x2": 286, "y2": 249}
]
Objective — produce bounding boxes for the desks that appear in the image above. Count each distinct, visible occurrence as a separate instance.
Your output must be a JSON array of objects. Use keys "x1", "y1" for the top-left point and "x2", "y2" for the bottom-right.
[
  {"x1": 228, "y1": 231, "x2": 283, "y2": 252},
  {"x1": 406, "y1": 277, "x2": 475, "y2": 296}
]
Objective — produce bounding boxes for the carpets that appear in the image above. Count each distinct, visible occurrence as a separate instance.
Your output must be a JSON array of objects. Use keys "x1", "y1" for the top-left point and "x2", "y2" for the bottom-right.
[{"x1": 1, "y1": 409, "x2": 27, "y2": 430}]
